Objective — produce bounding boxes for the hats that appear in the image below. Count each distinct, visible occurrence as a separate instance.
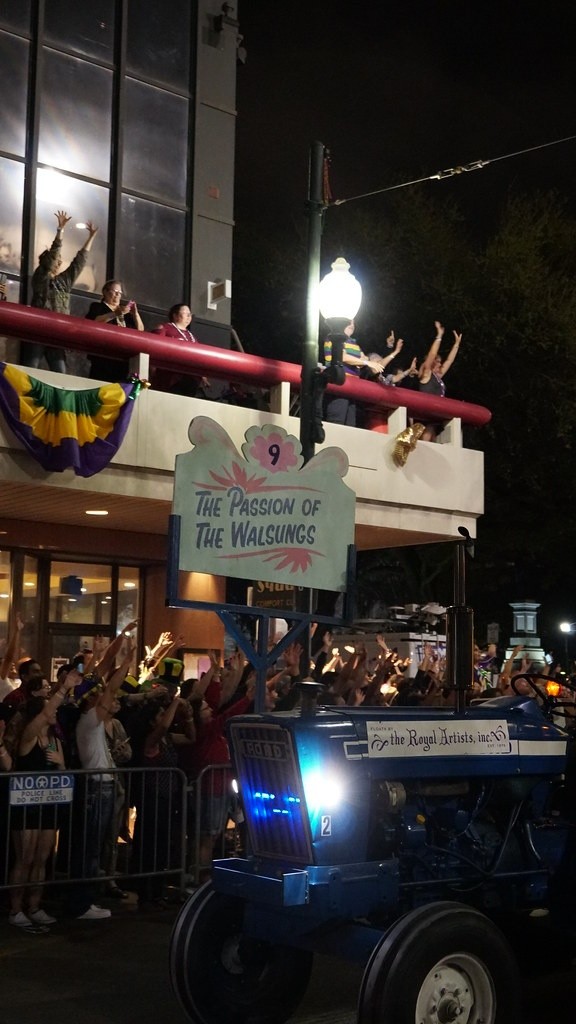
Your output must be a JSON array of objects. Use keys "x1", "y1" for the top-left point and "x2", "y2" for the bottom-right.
[
  {"x1": 120, "y1": 676, "x2": 140, "y2": 693},
  {"x1": 74, "y1": 679, "x2": 99, "y2": 705},
  {"x1": 152, "y1": 657, "x2": 185, "y2": 686}
]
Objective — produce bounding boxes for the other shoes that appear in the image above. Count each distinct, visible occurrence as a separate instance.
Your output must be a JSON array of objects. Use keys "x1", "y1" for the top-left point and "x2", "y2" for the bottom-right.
[
  {"x1": 28, "y1": 909, "x2": 57, "y2": 924},
  {"x1": 141, "y1": 898, "x2": 164, "y2": 912},
  {"x1": 90, "y1": 904, "x2": 104, "y2": 911},
  {"x1": 76, "y1": 908, "x2": 111, "y2": 919},
  {"x1": 105, "y1": 887, "x2": 128, "y2": 899},
  {"x1": 8, "y1": 912, "x2": 32, "y2": 926},
  {"x1": 152, "y1": 897, "x2": 178, "y2": 911}
]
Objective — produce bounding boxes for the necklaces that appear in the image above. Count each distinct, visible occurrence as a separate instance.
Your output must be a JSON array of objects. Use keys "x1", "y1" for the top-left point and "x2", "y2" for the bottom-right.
[{"x1": 171, "y1": 322, "x2": 195, "y2": 343}]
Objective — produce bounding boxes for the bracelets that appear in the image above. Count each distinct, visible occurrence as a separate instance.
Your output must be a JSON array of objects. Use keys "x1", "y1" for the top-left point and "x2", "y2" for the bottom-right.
[
  {"x1": 60, "y1": 687, "x2": 67, "y2": 694},
  {"x1": 55, "y1": 691, "x2": 64, "y2": 699},
  {"x1": 435, "y1": 337, "x2": 442, "y2": 341},
  {"x1": 389, "y1": 355, "x2": 395, "y2": 358}
]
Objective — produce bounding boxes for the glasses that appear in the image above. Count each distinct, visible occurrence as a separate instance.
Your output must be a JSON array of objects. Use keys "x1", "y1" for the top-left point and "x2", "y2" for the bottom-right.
[{"x1": 39, "y1": 685, "x2": 51, "y2": 689}]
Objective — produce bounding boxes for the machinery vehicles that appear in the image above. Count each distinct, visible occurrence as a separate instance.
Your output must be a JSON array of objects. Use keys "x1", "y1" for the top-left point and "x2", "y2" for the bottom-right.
[{"x1": 168, "y1": 539, "x2": 576, "y2": 1024}]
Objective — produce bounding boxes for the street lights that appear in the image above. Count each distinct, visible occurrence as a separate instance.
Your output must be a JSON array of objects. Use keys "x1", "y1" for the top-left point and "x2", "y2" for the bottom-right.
[{"x1": 295, "y1": 256, "x2": 363, "y2": 709}]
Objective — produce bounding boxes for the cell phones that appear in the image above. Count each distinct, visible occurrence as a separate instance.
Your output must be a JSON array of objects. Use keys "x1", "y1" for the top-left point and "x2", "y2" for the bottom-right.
[
  {"x1": 128, "y1": 300, "x2": 135, "y2": 311},
  {"x1": 48, "y1": 744, "x2": 56, "y2": 765}
]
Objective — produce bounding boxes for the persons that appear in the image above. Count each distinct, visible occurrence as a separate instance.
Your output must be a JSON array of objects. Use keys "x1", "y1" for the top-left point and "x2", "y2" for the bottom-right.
[
  {"x1": 19, "y1": 210, "x2": 98, "y2": 375},
  {"x1": 323, "y1": 317, "x2": 464, "y2": 441},
  {"x1": 85, "y1": 280, "x2": 144, "y2": 382},
  {"x1": 152, "y1": 305, "x2": 210, "y2": 397},
  {"x1": 0, "y1": 615, "x2": 576, "y2": 927}
]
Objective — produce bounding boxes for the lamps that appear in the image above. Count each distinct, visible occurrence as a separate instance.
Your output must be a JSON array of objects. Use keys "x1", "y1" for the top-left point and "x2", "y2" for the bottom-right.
[{"x1": 236, "y1": 33, "x2": 248, "y2": 64}]
